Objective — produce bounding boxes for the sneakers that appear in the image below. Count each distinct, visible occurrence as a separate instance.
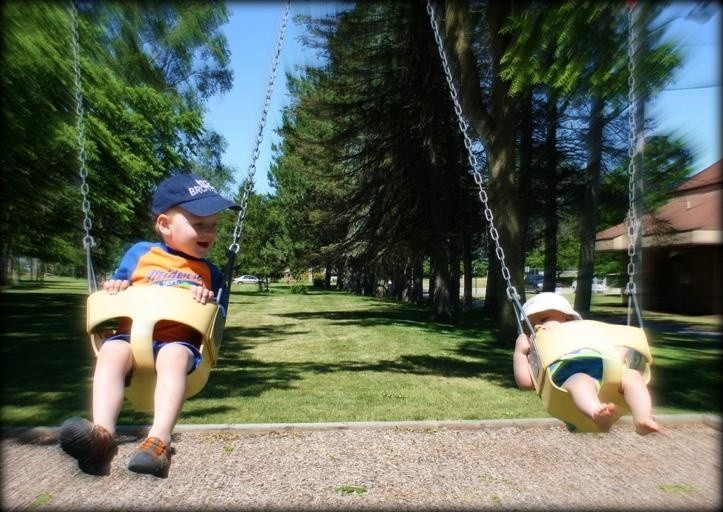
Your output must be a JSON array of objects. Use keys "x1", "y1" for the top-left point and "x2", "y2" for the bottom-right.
[
  {"x1": 59, "y1": 417, "x2": 118, "y2": 475},
  {"x1": 128, "y1": 437, "x2": 175, "y2": 477}
]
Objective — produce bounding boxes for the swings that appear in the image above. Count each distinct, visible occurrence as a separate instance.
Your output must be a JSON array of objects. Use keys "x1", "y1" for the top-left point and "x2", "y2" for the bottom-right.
[
  {"x1": 425, "y1": 0, "x2": 654, "y2": 430},
  {"x1": 69, "y1": 0, "x2": 291, "y2": 413}
]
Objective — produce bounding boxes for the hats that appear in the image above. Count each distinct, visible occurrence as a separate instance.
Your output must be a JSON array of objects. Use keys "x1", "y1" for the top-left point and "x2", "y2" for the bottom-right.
[
  {"x1": 152, "y1": 175, "x2": 242, "y2": 222},
  {"x1": 518, "y1": 292, "x2": 582, "y2": 335}
]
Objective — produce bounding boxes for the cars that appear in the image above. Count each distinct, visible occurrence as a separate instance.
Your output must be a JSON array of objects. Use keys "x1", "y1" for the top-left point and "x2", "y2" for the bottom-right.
[
  {"x1": 572, "y1": 278, "x2": 610, "y2": 294},
  {"x1": 525, "y1": 275, "x2": 561, "y2": 293},
  {"x1": 233, "y1": 275, "x2": 269, "y2": 285}
]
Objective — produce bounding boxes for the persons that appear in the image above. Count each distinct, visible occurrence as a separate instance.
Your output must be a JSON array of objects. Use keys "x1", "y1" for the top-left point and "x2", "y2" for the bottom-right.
[
  {"x1": 60, "y1": 174, "x2": 243, "y2": 477},
  {"x1": 512, "y1": 291, "x2": 660, "y2": 436}
]
